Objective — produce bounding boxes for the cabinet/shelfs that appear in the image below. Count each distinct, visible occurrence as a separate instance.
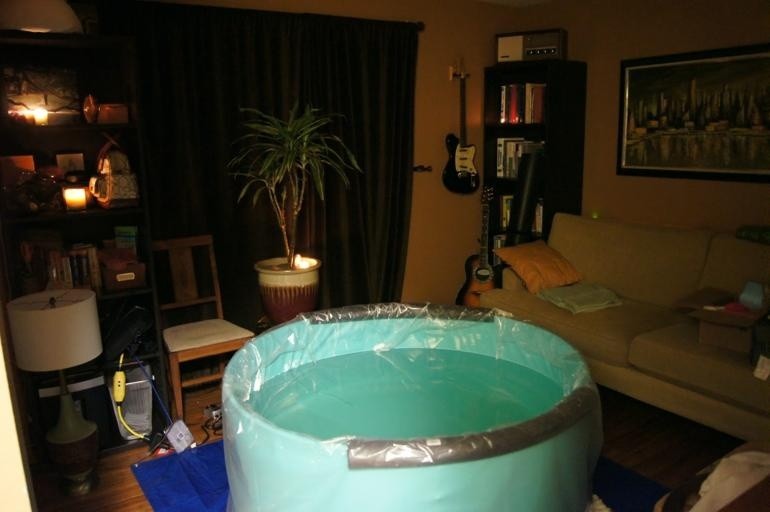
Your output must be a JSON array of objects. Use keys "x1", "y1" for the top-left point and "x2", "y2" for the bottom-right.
[
  {"x1": 482, "y1": 61, "x2": 587, "y2": 274},
  {"x1": 0, "y1": 36, "x2": 171, "y2": 471}
]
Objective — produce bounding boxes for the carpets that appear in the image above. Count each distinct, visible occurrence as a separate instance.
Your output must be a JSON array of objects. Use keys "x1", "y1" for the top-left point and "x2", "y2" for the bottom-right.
[{"x1": 130, "y1": 437, "x2": 674, "y2": 511}]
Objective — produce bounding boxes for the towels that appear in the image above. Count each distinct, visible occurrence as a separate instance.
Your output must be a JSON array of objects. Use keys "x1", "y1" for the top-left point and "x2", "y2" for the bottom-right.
[{"x1": 537, "y1": 281, "x2": 624, "y2": 315}]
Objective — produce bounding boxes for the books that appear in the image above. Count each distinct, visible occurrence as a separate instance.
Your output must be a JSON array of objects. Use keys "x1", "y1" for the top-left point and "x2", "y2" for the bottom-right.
[
  {"x1": 537, "y1": 277, "x2": 611, "y2": 308},
  {"x1": 16, "y1": 240, "x2": 102, "y2": 300},
  {"x1": 488, "y1": 80, "x2": 550, "y2": 273},
  {"x1": 564, "y1": 292, "x2": 623, "y2": 315}
]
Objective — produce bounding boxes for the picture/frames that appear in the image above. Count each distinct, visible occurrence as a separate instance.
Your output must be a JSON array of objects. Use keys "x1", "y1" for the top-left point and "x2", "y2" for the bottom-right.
[{"x1": 615, "y1": 43, "x2": 769, "y2": 182}]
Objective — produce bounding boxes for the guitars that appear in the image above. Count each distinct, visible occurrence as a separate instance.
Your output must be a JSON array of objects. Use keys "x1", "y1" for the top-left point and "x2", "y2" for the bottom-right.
[
  {"x1": 455, "y1": 182, "x2": 505, "y2": 310},
  {"x1": 442, "y1": 56, "x2": 480, "y2": 194}
]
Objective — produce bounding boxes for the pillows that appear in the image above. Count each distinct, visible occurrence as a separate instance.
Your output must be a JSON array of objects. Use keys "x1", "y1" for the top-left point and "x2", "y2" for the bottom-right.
[{"x1": 490, "y1": 240, "x2": 583, "y2": 296}]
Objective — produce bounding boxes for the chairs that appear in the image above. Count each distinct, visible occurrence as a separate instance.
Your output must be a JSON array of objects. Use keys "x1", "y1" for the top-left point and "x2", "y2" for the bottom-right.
[{"x1": 151, "y1": 234, "x2": 255, "y2": 419}]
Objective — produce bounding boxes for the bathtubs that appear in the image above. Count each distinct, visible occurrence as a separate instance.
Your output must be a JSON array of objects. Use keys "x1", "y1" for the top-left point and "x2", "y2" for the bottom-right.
[{"x1": 222, "y1": 310, "x2": 603, "y2": 512}]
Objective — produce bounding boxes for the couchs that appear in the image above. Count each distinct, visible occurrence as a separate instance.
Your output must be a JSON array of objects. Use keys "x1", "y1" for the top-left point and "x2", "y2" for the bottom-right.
[{"x1": 478, "y1": 212, "x2": 770, "y2": 441}]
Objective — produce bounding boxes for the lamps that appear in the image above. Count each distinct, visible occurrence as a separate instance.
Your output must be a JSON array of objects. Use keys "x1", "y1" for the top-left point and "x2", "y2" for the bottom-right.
[
  {"x1": 4, "y1": 290, "x2": 104, "y2": 495},
  {"x1": 1, "y1": 1, "x2": 83, "y2": 36}
]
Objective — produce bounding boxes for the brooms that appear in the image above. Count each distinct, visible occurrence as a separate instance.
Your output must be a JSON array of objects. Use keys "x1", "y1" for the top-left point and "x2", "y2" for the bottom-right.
[{"x1": 137, "y1": 357, "x2": 195, "y2": 454}]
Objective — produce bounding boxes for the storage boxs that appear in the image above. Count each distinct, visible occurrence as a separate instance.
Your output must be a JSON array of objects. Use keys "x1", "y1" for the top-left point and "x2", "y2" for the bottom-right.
[{"x1": 673, "y1": 281, "x2": 770, "y2": 354}]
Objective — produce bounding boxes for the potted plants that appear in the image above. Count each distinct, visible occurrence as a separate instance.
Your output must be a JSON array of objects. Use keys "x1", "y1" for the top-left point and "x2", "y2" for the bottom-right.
[{"x1": 224, "y1": 100, "x2": 364, "y2": 323}]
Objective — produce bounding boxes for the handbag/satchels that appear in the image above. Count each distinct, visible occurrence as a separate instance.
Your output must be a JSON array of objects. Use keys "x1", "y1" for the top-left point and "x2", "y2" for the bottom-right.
[{"x1": 89, "y1": 151, "x2": 141, "y2": 210}]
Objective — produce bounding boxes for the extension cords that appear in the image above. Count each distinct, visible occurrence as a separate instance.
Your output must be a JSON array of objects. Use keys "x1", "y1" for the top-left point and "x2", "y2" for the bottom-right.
[{"x1": 212, "y1": 408, "x2": 222, "y2": 420}]
